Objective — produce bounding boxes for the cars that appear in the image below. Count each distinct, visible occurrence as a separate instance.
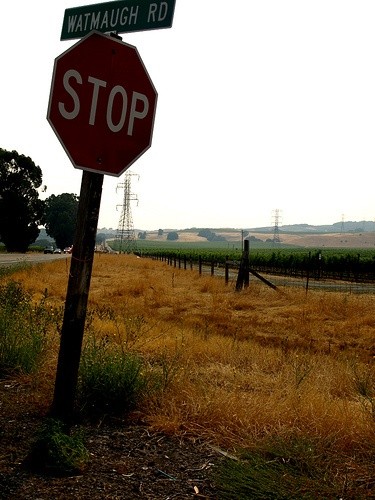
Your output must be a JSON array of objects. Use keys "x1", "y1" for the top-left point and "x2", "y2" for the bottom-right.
[
  {"x1": 44, "y1": 245, "x2": 54, "y2": 254},
  {"x1": 63, "y1": 247, "x2": 71, "y2": 253},
  {"x1": 54, "y1": 248, "x2": 61, "y2": 254}
]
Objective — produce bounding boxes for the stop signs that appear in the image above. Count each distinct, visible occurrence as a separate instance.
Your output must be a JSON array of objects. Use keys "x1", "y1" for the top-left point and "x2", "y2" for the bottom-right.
[{"x1": 44, "y1": 30, "x2": 158, "y2": 179}]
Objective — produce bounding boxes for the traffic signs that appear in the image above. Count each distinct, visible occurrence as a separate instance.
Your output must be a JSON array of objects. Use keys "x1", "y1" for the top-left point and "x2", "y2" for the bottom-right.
[{"x1": 60, "y1": 0, "x2": 176, "y2": 41}]
